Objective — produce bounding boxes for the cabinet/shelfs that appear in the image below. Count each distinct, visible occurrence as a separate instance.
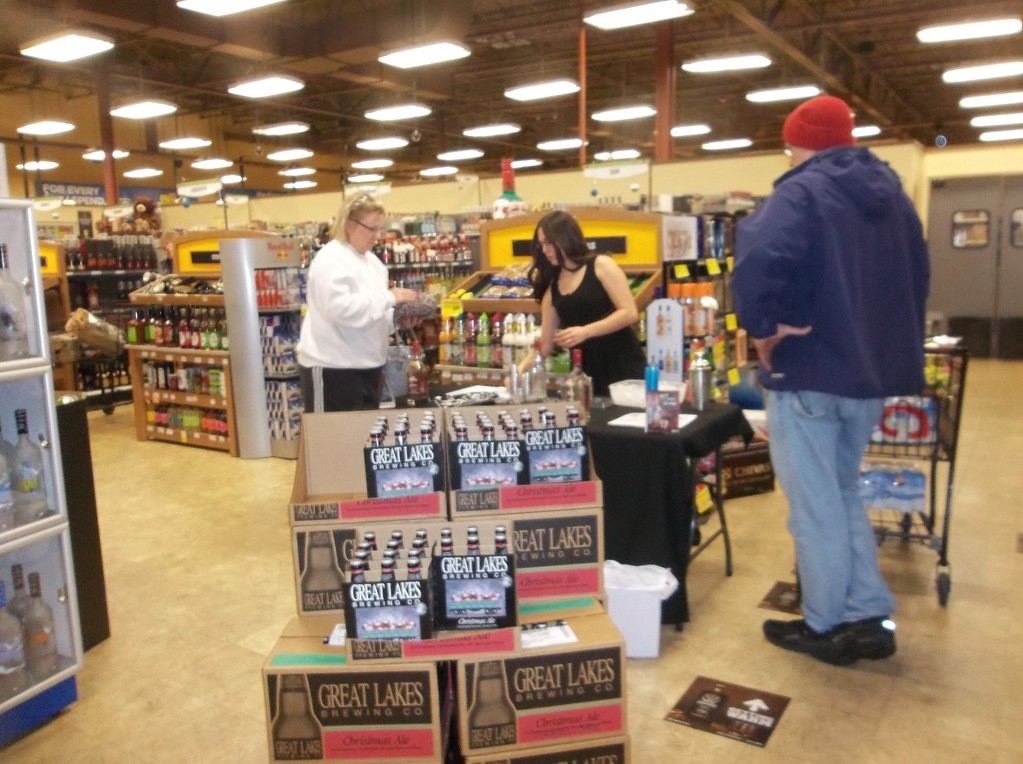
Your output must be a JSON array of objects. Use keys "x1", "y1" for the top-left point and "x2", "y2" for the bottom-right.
[
  {"x1": 41, "y1": 228, "x2": 475, "y2": 461},
  {"x1": 434, "y1": 207, "x2": 760, "y2": 385},
  {"x1": 0, "y1": 198, "x2": 85, "y2": 752}
]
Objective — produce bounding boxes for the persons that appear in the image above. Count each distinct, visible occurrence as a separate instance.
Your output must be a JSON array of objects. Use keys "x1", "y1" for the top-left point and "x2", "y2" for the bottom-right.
[
  {"x1": 731, "y1": 97, "x2": 932, "y2": 664},
  {"x1": 296, "y1": 190, "x2": 420, "y2": 413},
  {"x1": 503, "y1": 210, "x2": 648, "y2": 400}
]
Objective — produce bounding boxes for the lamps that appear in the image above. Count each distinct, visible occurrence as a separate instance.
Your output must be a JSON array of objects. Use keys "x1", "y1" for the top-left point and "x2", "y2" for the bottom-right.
[
  {"x1": 107, "y1": 63, "x2": 179, "y2": 120},
  {"x1": 362, "y1": 75, "x2": 432, "y2": 122},
  {"x1": 580, "y1": 0, "x2": 696, "y2": 32},
  {"x1": 681, "y1": 52, "x2": 772, "y2": 72},
  {"x1": 462, "y1": 97, "x2": 522, "y2": 138},
  {"x1": 227, "y1": 32, "x2": 304, "y2": 99},
  {"x1": 591, "y1": 61, "x2": 658, "y2": 121},
  {"x1": 251, "y1": 107, "x2": 310, "y2": 136},
  {"x1": 376, "y1": 0, "x2": 471, "y2": 68},
  {"x1": 17, "y1": 0, "x2": 116, "y2": 63},
  {"x1": 502, "y1": 34, "x2": 581, "y2": 104}
]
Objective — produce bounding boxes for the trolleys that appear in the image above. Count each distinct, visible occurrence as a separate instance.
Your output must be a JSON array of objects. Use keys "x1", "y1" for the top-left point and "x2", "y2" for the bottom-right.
[
  {"x1": 796, "y1": 336, "x2": 970, "y2": 608},
  {"x1": 51, "y1": 309, "x2": 133, "y2": 416}
]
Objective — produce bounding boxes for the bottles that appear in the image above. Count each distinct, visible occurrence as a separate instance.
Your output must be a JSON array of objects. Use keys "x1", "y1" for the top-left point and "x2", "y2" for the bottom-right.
[
  {"x1": 374, "y1": 225, "x2": 473, "y2": 298},
  {"x1": 406, "y1": 341, "x2": 430, "y2": 405},
  {"x1": 369, "y1": 405, "x2": 582, "y2": 453},
  {"x1": 507, "y1": 364, "x2": 521, "y2": 406},
  {"x1": 75, "y1": 358, "x2": 133, "y2": 389},
  {"x1": 68, "y1": 236, "x2": 158, "y2": 309},
  {"x1": 689, "y1": 352, "x2": 711, "y2": 411},
  {"x1": 389, "y1": 319, "x2": 437, "y2": 346},
  {"x1": 564, "y1": 349, "x2": 591, "y2": 425},
  {"x1": 646, "y1": 358, "x2": 659, "y2": 391},
  {"x1": 91, "y1": 310, "x2": 144, "y2": 332},
  {"x1": 520, "y1": 372, "x2": 534, "y2": 403},
  {"x1": 0, "y1": 243, "x2": 29, "y2": 358},
  {"x1": 348, "y1": 526, "x2": 508, "y2": 582},
  {"x1": 0, "y1": 564, "x2": 58, "y2": 701},
  {"x1": 531, "y1": 352, "x2": 547, "y2": 398},
  {"x1": 0, "y1": 409, "x2": 48, "y2": 528},
  {"x1": 128, "y1": 304, "x2": 237, "y2": 436}
]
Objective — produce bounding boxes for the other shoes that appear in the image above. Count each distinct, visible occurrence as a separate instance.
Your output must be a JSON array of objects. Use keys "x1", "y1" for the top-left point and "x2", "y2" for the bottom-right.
[{"x1": 762, "y1": 617, "x2": 896, "y2": 664}]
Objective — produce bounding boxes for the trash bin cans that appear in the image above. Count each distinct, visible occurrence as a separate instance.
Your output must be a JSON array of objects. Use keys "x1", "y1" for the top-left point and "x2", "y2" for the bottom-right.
[
  {"x1": 605, "y1": 565, "x2": 664, "y2": 662},
  {"x1": 53, "y1": 390, "x2": 112, "y2": 654}
]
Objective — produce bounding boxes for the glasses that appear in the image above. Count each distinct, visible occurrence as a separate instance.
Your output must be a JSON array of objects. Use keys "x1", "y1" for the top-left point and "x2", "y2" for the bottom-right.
[
  {"x1": 534, "y1": 238, "x2": 554, "y2": 248},
  {"x1": 356, "y1": 220, "x2": 383, "y2": 233},
  {"x1": 783, "y1": 144, "x2": 796, "y2": 157}
]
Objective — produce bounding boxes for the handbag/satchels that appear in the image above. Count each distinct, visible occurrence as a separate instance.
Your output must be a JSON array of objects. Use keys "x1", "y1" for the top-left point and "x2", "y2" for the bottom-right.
[{"x1": 65, "y1": 306, "x2": 128, "y2": 358}]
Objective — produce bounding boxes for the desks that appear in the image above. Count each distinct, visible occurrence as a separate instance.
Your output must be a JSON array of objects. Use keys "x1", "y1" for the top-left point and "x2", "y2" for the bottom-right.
[{"x1": 396, "y1": 378, "x2": 754, "y2": 632}]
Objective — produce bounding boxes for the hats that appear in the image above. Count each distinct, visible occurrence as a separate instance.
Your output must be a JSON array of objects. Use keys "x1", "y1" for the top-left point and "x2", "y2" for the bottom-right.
[{"x1": 781, "y1": 95, "x2": 857, "y2": 150}]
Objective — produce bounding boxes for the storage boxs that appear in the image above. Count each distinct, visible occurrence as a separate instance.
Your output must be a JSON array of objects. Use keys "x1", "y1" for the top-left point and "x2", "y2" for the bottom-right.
[
  {"x1": 698, "y1": 439, "x2": 775, "y2": 501},
  {"x1": 266, "y1": 400, "x2": 631, "y2": 764}
]
